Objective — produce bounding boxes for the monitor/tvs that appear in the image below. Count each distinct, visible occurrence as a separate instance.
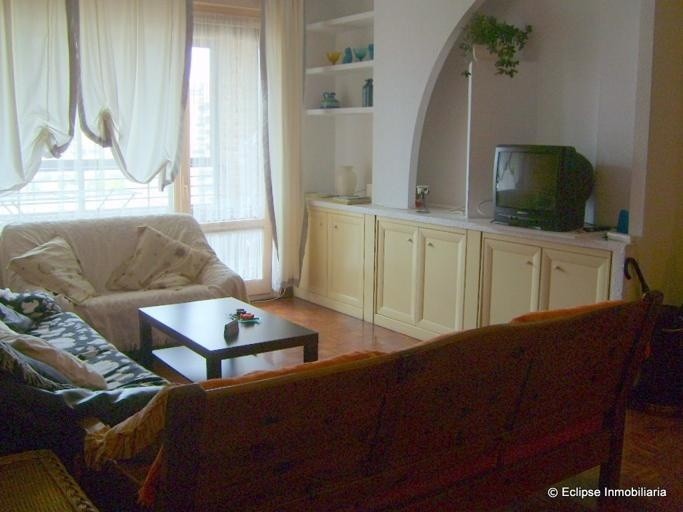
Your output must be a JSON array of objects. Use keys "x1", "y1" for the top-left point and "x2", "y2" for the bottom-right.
[{"x1": 492, "y1": 143, "x2": 595, "y2": 233}]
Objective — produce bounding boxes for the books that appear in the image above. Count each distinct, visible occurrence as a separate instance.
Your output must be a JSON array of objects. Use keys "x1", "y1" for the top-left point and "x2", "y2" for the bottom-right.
[{"x1": 327, "y1": 192, "x2": 371, "y2": 205}]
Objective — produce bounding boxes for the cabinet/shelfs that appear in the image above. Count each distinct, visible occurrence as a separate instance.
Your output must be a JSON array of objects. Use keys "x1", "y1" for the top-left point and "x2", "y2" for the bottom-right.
[{"x1": 302, "y1": 9, "x2": 375, "y2": 117}]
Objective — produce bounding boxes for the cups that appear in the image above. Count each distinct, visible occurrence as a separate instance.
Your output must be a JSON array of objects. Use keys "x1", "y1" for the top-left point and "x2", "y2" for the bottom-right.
[
  {"x1": 413, "y1": 184, "x2": 432, "y2": 213},
  {"x1": 324, "y1": 43, "x2": 373, "y2": 66}
]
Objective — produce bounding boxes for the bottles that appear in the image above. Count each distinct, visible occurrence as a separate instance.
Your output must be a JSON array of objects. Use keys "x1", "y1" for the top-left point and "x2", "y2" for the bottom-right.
[
  {"x1": 361, "y1": 78, "x2": 373, "y2": 108},
  {"x1": 335, "y1": 165, "x2": 357, "y2": 198},
  {"x1": 318, "y1": 92, "x2": 340, "y2": 108}
]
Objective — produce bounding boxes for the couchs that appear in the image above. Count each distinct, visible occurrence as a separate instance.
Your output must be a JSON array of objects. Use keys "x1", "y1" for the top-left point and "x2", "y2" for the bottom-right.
[{"x1": 73, "y1": 287, "x2": 666, "y2": 512}]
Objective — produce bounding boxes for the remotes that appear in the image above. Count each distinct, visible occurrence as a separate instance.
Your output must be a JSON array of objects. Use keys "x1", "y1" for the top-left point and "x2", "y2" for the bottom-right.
[{"x1": 583, "y1": 225, "x2": 613, "y2": 233}]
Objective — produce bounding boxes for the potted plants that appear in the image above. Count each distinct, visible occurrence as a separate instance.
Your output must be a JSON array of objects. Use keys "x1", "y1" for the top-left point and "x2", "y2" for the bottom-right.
[{"x1": 458, "y1": 12, "x2": 535, "y2": 82}]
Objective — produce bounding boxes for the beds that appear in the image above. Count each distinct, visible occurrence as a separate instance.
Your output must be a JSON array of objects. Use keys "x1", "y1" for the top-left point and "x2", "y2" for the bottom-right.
[{"x1": 135, "y1": 293, "x2": 320, "y2": 385}]
